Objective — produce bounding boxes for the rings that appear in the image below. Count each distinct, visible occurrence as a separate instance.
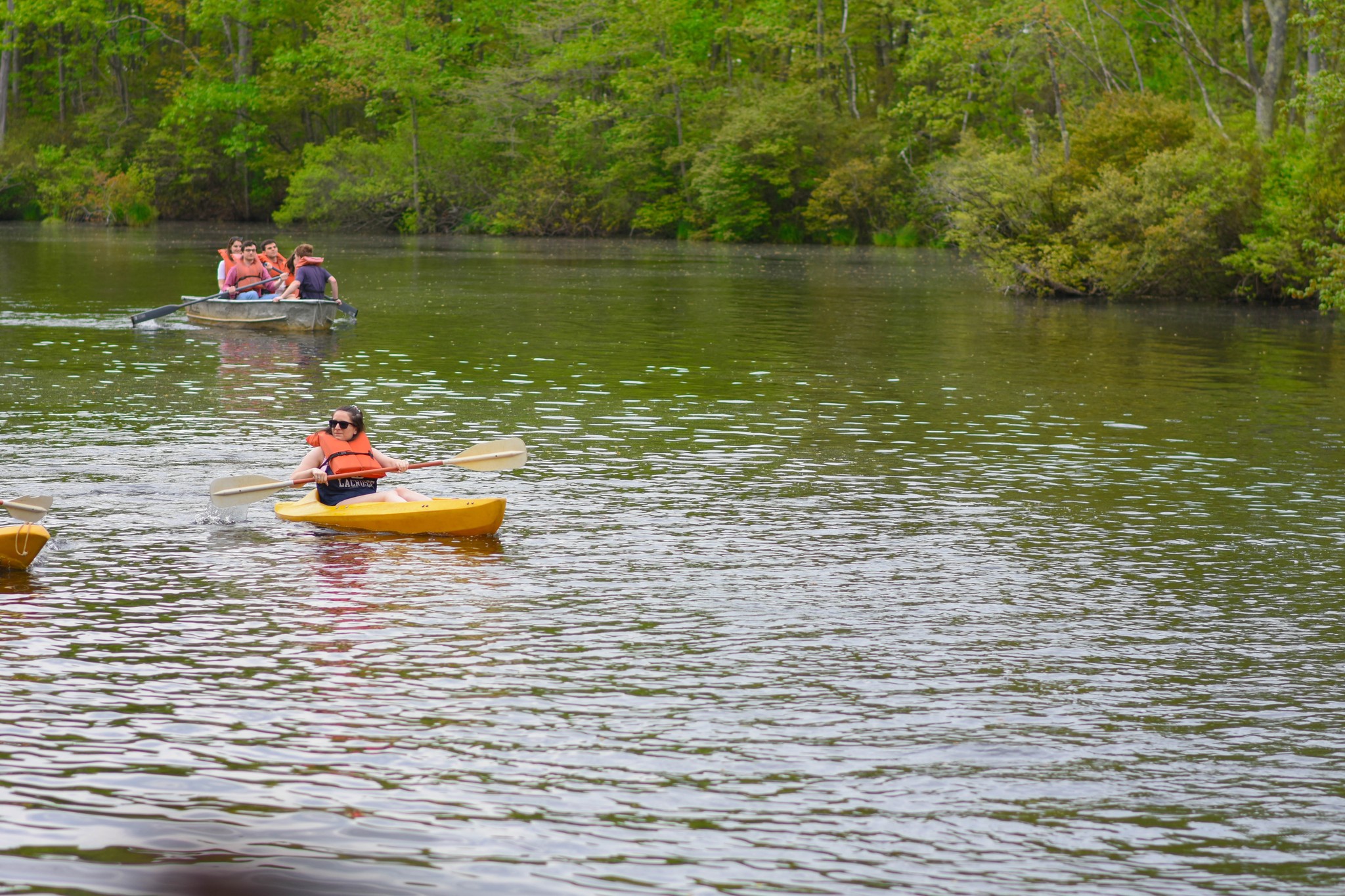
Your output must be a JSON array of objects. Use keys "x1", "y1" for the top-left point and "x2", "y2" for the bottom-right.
[{"x1": 317, "y1": 477, "x2": 321, "y2": 479}]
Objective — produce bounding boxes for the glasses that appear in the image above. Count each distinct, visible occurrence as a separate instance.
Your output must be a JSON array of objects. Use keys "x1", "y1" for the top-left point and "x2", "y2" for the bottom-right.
[
  {"x1": 244, "y1": 249, "x2": 256, "y2": 251},
  {"x1": 229, "y1": 237, "x2": 243, "y2": 244},
  {"x1": 329, "y1": 420, "x2": 357, "y2": 429}
]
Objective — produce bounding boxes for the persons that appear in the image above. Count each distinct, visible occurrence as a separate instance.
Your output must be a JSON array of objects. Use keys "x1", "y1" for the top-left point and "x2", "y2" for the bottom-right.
[
  {"x1": 217, "y1": 236, "x2": 342, "y2": 305},
  {"x1": 289, "y1": 405, "x2": 432, "y2": 506}
]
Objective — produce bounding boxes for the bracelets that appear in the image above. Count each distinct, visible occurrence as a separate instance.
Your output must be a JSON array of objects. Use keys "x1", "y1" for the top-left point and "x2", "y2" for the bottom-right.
[{"x1": 332, "y1": 298, "x2": 338, "y2": 301}]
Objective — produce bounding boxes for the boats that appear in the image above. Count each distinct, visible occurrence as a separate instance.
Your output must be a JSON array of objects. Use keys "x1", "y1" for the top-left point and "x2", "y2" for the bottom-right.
[
  {"x1": 272, "y1": 486, "x2": 507, "y2": 536},
  {"x1": 0, "y1": 522, "x2": 51, "y2": 572},
  {"x1": 180, "y1": 294, "x2": 341, "y2": 332}
]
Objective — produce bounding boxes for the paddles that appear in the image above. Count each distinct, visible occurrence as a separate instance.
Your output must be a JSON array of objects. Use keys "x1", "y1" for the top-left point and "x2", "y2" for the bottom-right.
[
  {"x1": 0, "y1": 494, "x2": 54, "y2": 522},
  {"x1": 131, "y1": 277, "x2": 281, "y2": 328},
  {"x1": 208, "y1": 439, "x2": 527, "y2": 508},
  {"x1": 263, "y1": 261, "x2": 358, "y2": 318}
]
